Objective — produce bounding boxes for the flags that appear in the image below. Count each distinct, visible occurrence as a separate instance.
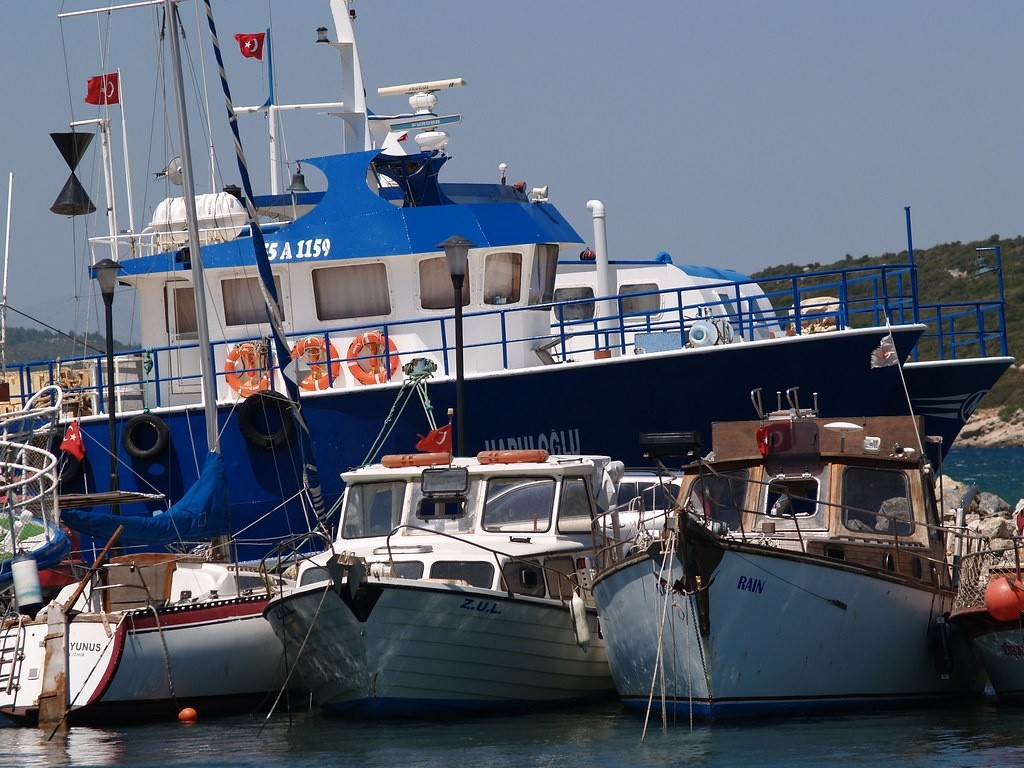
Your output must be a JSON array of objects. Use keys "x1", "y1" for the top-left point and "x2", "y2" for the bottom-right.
[
  {"x1": 415, "y1": 424, "x2": 453, "y2": 455},
  {"x1": 84, "y1": 73, "x2": 119, "y2": 105},
  {"x1": 59, "y1": 419, "x2": 86, "y2": 462},
  {"x1": 234, "y1": 33, "x2": 267, "y2": 61},
  {"x1": 756, "y1": 420, "x2": 793, "y2": 456}
]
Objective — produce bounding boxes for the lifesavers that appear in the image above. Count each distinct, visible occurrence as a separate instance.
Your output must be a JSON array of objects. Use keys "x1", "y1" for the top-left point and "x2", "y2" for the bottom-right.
[
  {"x1": 476, "y1": 449, "x2": 550, "y2": 465},
  {"x1": 26, "y1": 427, "x2": 83, "y2": 486},
  {"x1": 238, "y1": 388, "x2": 296, "y2": 452},
  {"x1": 224, "y1": 342, "x2": 277, "y2": 399},
  {"x1": 346, "y1": 330, "x2": 400, "y2": 385},
  {"x1": 290, "y1": 336, "x2": 341, "y2": 391},
  {"x1": 380, "y1": 452, "x2": 454, "y2": 469},
  {"x1": 121, "y1": 414, "x2": 170, "y2": 459}
]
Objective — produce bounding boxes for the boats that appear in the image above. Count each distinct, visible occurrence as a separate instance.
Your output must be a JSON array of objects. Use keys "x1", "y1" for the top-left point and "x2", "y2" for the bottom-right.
[{"x1": 0, "y1": 0, "x2": 1024, "y2": 726}]
